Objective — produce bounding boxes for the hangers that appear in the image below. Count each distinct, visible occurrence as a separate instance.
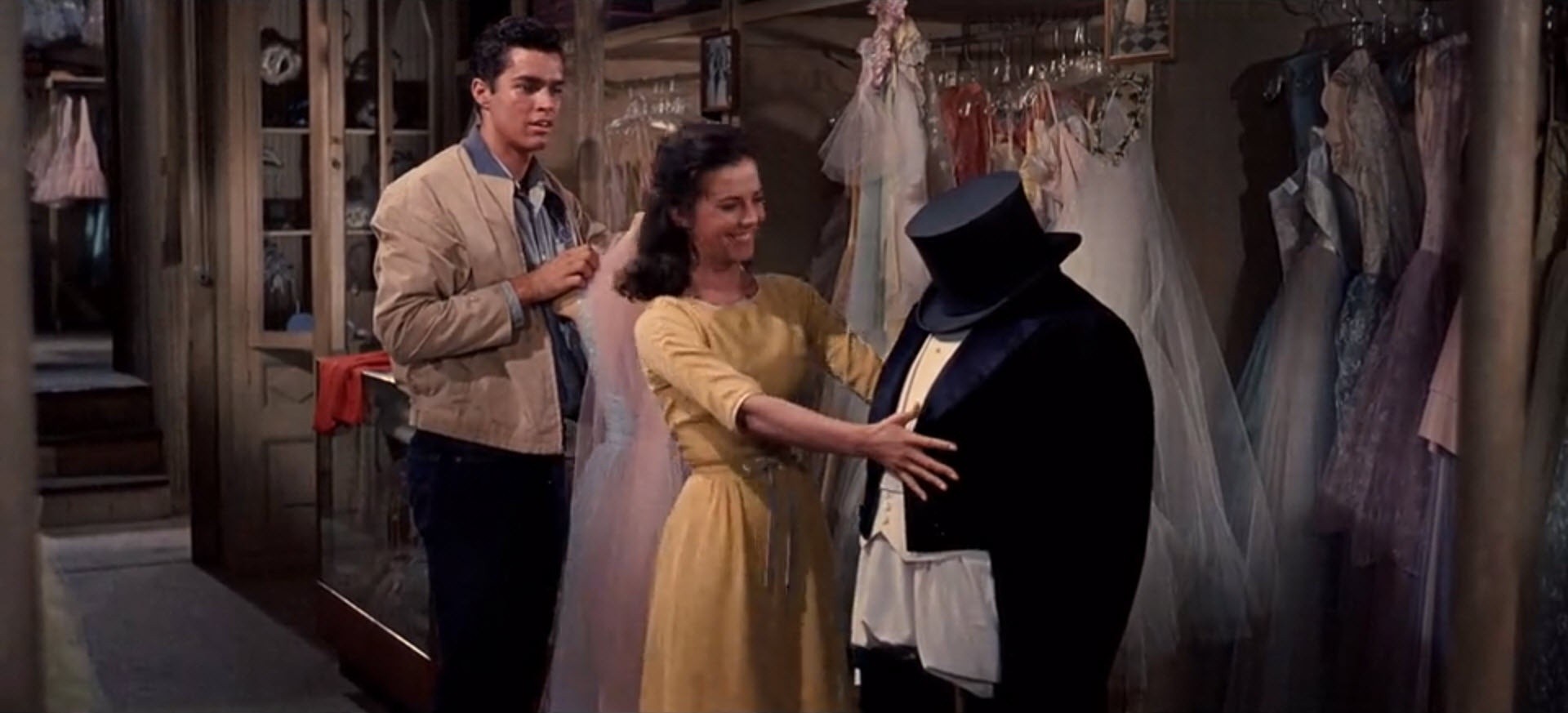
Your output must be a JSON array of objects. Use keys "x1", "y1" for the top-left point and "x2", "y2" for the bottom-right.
[
  {"x1": 599, "y1": 76, "x2": 690, "y2": 133},
  {"x1": 1260, "y1": 0, "x2": 1560, "y2": 102},
  {"x1": 929, "y1": 13, "x2": 1151, "y2": 115}
]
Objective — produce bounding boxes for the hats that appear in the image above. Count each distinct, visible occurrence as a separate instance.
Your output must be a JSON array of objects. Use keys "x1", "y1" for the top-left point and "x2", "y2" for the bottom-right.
[{"x1": 902, "y1": 171, "x2": 1082, "y2": 331}]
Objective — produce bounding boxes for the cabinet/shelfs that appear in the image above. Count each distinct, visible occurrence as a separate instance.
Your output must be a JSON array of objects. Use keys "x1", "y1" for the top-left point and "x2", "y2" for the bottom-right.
[{"x1": 208, "y1": 0, "x2": 458, "y2": 566}]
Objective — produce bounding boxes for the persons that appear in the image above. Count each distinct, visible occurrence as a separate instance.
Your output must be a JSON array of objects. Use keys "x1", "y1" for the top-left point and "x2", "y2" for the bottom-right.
[
  {"x1": 609, "y1": 122, "x2": 957, "y2": 713},
  {"x1": 851, "y1": 170, "x2": 1154, "y2": 713},
  {"x1": 371, "y1": 16, "x2": 633, "y2": 713}
]
